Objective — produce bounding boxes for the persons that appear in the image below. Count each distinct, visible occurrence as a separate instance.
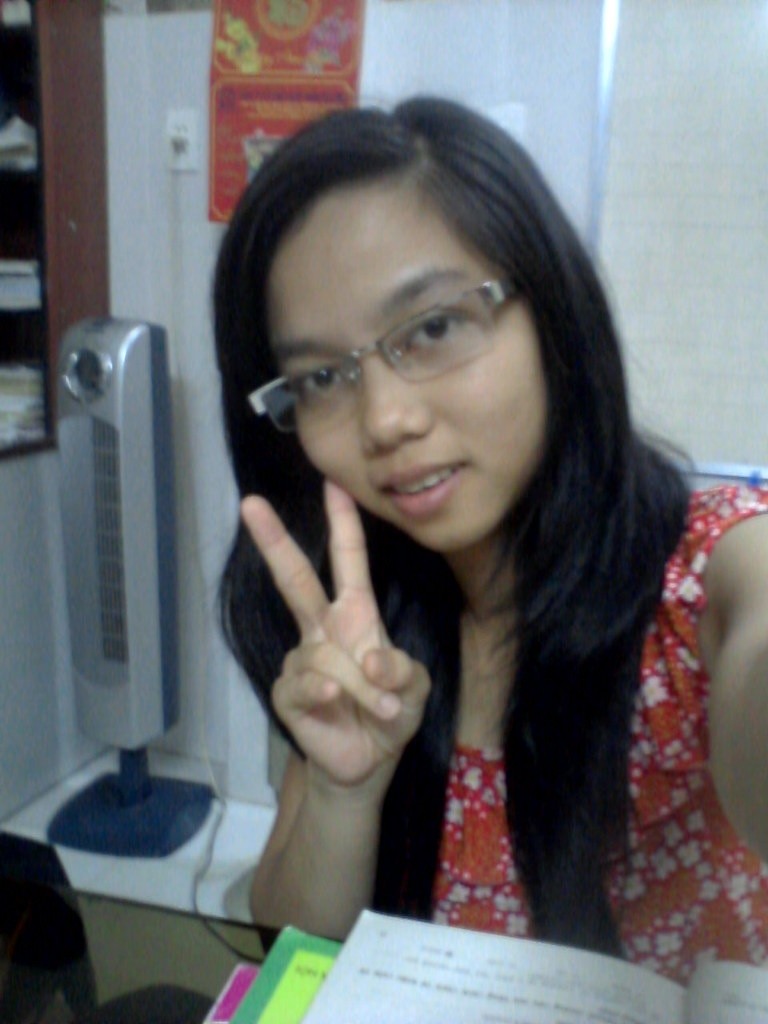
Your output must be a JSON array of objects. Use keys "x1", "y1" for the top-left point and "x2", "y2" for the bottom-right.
[{"x1": 214, "y1": 97, "x2": 768, "y2": 982}]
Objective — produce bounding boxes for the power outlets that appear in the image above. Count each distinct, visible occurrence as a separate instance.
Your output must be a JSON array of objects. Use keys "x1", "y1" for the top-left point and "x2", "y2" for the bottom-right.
[{"x1": 166, "y1": 110, "x2": 201, "y2": 174}]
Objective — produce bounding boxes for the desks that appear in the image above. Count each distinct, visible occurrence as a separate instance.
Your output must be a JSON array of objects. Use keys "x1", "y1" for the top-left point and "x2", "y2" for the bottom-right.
[{"x1": 0, "y1": 744, "x2": 278, "y2": 931}]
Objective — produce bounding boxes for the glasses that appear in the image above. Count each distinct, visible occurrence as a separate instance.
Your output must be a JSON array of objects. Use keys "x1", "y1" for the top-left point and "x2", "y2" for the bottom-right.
[{"x1": 248, "y1": 278, "x2": 517, "y2": 431}]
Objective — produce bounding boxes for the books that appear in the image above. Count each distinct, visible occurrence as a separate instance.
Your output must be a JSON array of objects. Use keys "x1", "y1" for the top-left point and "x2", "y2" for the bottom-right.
[{"x1": 206, "y1": 909, "x2": 768, "y2": 1023}]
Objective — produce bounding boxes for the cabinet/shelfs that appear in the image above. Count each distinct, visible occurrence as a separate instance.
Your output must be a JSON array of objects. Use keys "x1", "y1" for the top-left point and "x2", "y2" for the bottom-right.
[{"x1": 0, "y1": 0, "x2": 111, "y2": 462}]
